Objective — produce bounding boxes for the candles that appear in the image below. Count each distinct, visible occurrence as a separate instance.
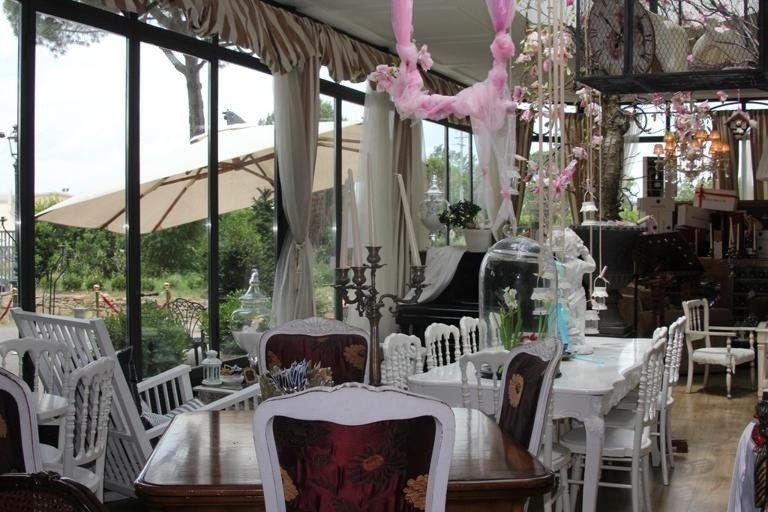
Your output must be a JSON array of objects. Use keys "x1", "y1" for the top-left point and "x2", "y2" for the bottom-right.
[{"x1": 338, "y1": 152, "x2": 423, "y2": 267}]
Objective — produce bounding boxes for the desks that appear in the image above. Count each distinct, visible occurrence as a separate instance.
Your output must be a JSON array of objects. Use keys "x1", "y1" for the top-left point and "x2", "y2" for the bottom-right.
[
  {"x1": 192, "y1": 366, "x2": 259, "y2": 404},
  {"x1": 401, "y1": 328, "x2": 667, "y2": 509},
  {"x1": 130, "y1": 404, "x2": 556, "y2": 508}
]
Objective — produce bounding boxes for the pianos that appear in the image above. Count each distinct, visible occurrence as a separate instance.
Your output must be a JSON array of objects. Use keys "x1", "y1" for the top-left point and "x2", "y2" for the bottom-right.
[{"x1": 396, "y1": 245, "x2": 543, "y2": 373}]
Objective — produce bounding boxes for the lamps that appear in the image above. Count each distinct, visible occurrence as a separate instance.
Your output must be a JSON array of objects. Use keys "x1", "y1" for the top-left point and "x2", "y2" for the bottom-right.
[{"x1": 653, "y1": 92, "x2": 733, "y2": 183}]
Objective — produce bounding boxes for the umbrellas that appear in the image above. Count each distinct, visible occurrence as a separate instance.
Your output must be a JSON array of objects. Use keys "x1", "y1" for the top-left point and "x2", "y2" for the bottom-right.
[{"x1": 33, "y1": 120, "x2": 365, "y2": 233}]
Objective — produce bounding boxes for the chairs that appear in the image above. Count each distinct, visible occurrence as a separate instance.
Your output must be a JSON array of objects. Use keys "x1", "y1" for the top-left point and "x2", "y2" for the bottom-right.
[
  {"x1": 249, "y1": 381, "x2": 451, "y2": 512},
  {"x1": 0, "y1": 366, "x2": 50, "y2": 479},
  {"x1": 2, "y1": 471, "x2": 134, "y2": 512},
  {"x1": 486, "y1": 337, "x2": 560, "y2": 508},
  {"x1": 10, "y1": 385, "x2": 69, "y2": 428},
  {"x1": 32, "y1": 358, "x2": 117, "y2": 505},
  {"x1": 456, "y1": 312, "x2": 486, "y2": 352},
  {"x1": 9, "y1": 306, "x2": 263, "y2": 497},
  {"x1": 556, "y1": 338, "x2": 669, "y2": 510},
  {"x1": 0, "y1": 336, "x2": 74, "y2": 474},
  {"x1": 490, "y1": 308, "x2": 508, "y2": 343},
  {"x1": 421, "y1": 322, "x2": 460, "y2": 372},
  {"x1": 570, "y1": 324, "x2": 671, "y2": 487},
  {"x1": 679, "y1": 297, "x2": 754, "y2": 398},
  {"x1": 454, "y1": 350, "x2": 576, "y2": 510},
  {"x1": 618, "y1": 315, "x2": 690, "y2": 480},
  {"x1": 253, "y1": 317, "x2": 373, "y2": 400},
  {"x1": 377, "y1": 331, "x2": 422, "y2": 387}
]
